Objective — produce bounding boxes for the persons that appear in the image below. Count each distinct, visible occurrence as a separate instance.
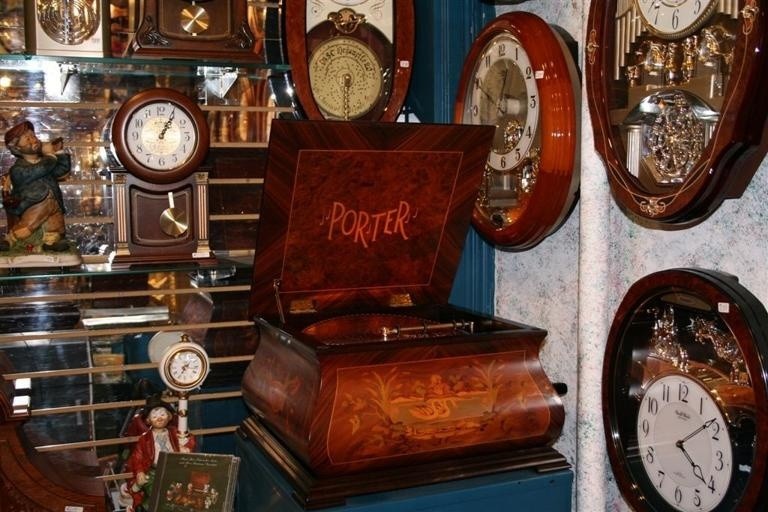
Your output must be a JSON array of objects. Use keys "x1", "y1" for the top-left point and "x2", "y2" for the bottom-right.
[
  {"x1": 0, "y1": 121, "x2": 71, "y2": 252},
  {"x1": 131, "y1": 397, "x2": 195, "y2": 512}
]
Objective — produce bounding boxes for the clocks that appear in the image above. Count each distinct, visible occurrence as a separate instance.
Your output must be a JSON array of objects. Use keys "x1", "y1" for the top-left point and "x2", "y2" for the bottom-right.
[
  {"x1": 582, "y1": 1, "x2": 766, "y2": 226},
  {"x1": 103, "y1": 87, "x2": 221, "y2": 269},
  {"x1": 601, "y1": 266, "x2": 766, "y2": 510},
  {"x1": 160, "y1": 336, "x2": 211, "y2": 395},
  {"x1": 283, "y1": 0, "x2": 416, "y2": 122},
  {"x1": 452, "y1": 10, "x2": 580, "y2": 252}
]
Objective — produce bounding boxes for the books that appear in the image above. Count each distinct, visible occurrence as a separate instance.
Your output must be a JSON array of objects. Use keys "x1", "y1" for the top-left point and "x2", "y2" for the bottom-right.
[{"x1": 148, "y1": 451, "x2": 242, "y2": 512}]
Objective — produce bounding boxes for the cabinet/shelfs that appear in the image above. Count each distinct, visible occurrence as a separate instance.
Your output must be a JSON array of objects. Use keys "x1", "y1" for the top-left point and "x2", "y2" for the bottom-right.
[{"x1": 0, "y1": 0, "x2": 435, "y2": 512}]
